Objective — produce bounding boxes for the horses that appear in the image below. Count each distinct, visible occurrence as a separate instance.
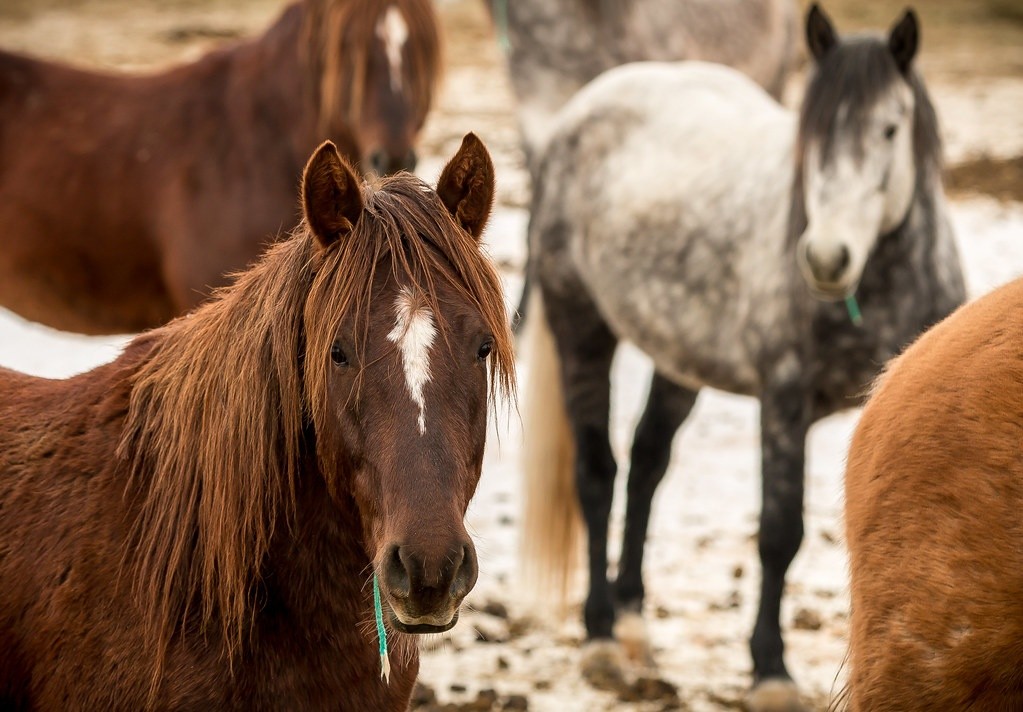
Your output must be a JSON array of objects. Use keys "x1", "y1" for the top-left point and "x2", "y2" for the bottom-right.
[{"x1": 0, "y1": 0, "x2": 1023, "y2": 712}]
